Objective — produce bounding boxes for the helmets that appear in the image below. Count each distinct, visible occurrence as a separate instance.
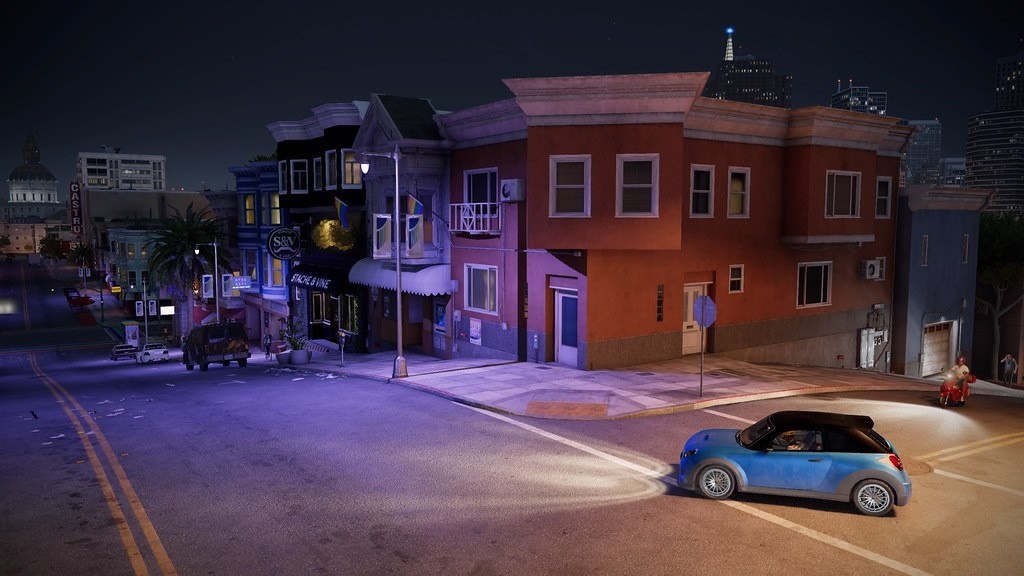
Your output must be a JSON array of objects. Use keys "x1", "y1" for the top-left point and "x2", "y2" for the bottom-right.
[{"x1": 957, "y1": 356, "x2": 965, "y2": 364}]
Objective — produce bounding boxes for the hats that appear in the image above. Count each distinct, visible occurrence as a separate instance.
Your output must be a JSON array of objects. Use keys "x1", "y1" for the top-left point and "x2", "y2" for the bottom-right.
[{"x1": 782, "y1": 431, "x2": 795, "y2": 439}]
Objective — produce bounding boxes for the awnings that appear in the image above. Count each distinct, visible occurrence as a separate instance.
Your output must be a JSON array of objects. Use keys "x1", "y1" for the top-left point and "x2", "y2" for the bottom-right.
[
  {"x1": 348, "y1": 257, "x2": 452, "y2": 297},
  {"x1": 286, "y1": 263, "x2": 360, "y2": 296}
]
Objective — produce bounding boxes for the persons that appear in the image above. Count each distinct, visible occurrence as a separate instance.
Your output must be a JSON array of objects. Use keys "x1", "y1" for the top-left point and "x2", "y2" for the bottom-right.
[
  {"x1": 163, "y1": 326, "x2": 170, "y2": 340},
  {"x1": 263, "y1": 329, "x2": 272, "y2": 359},
  {"x1": 944, "y1": 355, "x2": 970, "y2": 402},
  {"x1": 767, "y1": 431, "x2": 816, "y2": 451},
  {"x1": 1001, "y1": 353, "x2": 1018, "y2": 388}
]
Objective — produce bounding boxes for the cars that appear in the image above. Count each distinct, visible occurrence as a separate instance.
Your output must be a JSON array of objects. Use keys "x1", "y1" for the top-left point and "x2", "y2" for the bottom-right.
[
  {"x1": 676, "y1": 411, "x2": 912, "y2": 517},
  {"x1": 110, "y1": 344, "x2": 138, "y2": 361},
  {"x1": 63, "y1": 287, "x2": 80, "y2": 302},
  {"x1": 140, "y1": 343, "x2": 170, "y2": 364}
]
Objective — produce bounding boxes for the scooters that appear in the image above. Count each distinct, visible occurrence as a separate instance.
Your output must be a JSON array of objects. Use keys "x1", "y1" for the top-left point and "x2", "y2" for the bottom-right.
[{"x1": 940, "y1": 367, "x2": 976, "y2": 407}]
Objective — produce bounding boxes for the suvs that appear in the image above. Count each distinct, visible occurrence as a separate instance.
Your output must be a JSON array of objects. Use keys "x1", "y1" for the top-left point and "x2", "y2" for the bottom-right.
[{"x1": 182, "y1": 320, "x2": 251, "y2": 371}]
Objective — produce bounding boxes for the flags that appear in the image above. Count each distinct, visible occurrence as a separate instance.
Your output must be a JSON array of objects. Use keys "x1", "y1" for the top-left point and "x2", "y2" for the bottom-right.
[
  {"x1": 334, "y1": 196, "x2": 350, "y2": 230},
  {"x1": 407, "y1": 193, "x2": 423, "y2": 215}
]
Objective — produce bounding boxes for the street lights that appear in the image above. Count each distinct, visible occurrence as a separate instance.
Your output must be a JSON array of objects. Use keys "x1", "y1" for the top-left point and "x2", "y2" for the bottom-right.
[
  {"x1": 359, "y1": 144, "x2": 408, "y2": 378},
  {"x1": 194, "y1": 237, "x2": 219, "y2": 324},
  {"x1": 130, "y1": 277, "x2": 148, "y2": 343}
]
